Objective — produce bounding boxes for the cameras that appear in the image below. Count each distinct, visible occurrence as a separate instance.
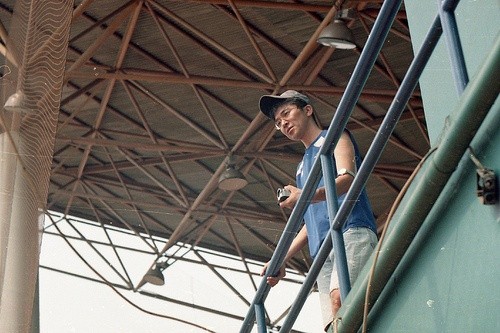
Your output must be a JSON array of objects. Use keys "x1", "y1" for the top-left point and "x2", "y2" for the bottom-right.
[{"x1": 277, "y1": 188, "x2": 290, "y2": 202}]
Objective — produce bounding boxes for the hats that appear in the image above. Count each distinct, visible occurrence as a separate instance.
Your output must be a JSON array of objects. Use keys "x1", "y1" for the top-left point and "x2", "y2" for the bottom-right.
[{"x1": 259, "y1": 90, "x2": 311, "y2": 118}]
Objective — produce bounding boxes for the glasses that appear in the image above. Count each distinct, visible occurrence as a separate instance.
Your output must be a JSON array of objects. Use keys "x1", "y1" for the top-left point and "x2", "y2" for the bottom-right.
[{"x1": 274, "y1": 107, "x2": 298, "y2": 130}]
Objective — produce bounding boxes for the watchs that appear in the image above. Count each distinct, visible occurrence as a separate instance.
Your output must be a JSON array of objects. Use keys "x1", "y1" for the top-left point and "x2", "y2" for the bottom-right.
[{"x1": 336, "y1": 167, "x2": 356, "y2": 177}]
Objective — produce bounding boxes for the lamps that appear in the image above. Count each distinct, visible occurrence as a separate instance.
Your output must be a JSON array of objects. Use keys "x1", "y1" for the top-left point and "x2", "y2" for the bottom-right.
[
  {"x1": 316, "y1": 3, "x2": 358, "y2": 50},
  {"x1": 218, "y1": 154, "x2": 248, "y2": 191},
  {"x1": 4, "y1": 83, "x2": 30, "y2": 113},
  {"x1": 143, "y1": 261, "x2": 169, "y2": 286}
]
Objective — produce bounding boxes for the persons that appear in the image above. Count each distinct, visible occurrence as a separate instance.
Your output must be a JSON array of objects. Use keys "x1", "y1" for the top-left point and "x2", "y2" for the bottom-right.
[{"x1": 259, "y1": 89, "x2": 379, "y2": 332}]
